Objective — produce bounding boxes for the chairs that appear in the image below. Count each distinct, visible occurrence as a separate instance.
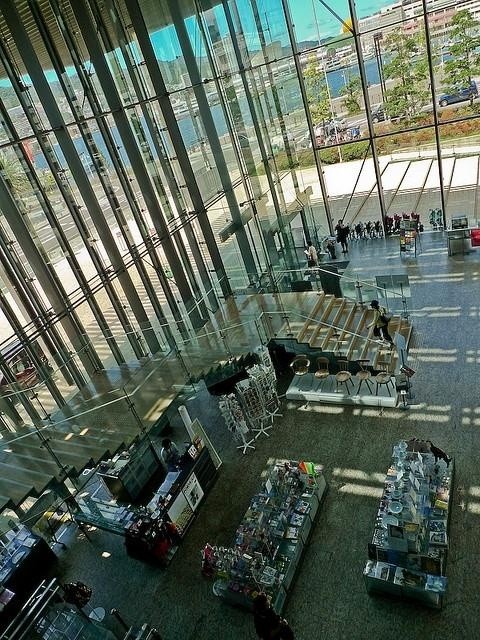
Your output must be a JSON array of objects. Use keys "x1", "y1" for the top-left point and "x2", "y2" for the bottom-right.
[{"x1": 288, "y1": 357, "x2": 394, "y2": 397}]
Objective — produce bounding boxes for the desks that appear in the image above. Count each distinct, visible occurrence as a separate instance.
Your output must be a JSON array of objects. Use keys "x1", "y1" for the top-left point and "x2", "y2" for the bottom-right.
[
  {"x1": 213, "y1": 456, "x2": 329, "y2": 616},
  {"x1": 71, "y1": 494, "x2": 138, "y2": 543},
  {"x1": 0, "y1": 533, "x2": 60, "y2": 626},
  {"x1": 362, "y1": 439, "x2": 456, "y2": 610}
]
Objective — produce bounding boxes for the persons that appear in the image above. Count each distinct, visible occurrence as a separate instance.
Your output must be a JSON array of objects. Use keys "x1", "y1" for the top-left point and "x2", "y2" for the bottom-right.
[
  {"x1": 306, "y1": 240, "x2": 320, "y2": 267},
  {"x1": 366, "y1": 300, "x2": 396, "y2": 350},
  {"x1": 335, "y1": 219, "x2": 349, "y2": 253},
  {"x1": 160, "y1": 438, "x2": 181, "y2": 471},
  {"x1": 252, "y1": 593, "x2": 294, "y2": 640}
]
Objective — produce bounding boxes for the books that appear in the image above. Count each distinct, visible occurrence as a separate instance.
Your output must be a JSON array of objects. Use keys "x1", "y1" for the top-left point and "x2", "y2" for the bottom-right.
[
  {"x1": 363, "y1": 438, "x2": 451, "y2": 594},
  {"x1": 234, "y1": 457, "x2": 318, "y2": 546}
]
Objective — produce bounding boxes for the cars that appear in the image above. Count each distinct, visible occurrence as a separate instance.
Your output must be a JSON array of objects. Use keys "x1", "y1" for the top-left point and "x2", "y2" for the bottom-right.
[
  {"x1": 370, "y1": 102, "x2": 405, "y2": 123},
  {"x1": 439, "y1": 80, "x2": 478, "y2": 106},
  {"x1": 307, "y1": 117, "x2": 348, "y2": 140}
]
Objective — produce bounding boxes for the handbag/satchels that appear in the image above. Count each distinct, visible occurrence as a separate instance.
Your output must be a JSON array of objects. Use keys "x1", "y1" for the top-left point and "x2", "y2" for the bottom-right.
[
  {"x1": 308, "y1": 259, "x2": 317, "y2": 267},
  {"x1": 380, "y1": 313, "x2": 390, "y2": 323}
]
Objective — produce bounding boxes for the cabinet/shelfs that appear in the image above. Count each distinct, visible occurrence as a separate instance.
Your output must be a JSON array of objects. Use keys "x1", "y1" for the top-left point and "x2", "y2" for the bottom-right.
[{"x1": 97, "y1": 432, "x2": 164, "y2": 507}]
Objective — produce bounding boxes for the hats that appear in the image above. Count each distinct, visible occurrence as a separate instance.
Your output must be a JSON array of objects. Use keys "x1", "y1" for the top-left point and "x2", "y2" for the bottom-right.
[{"x1": 369, "y1": 300, "x2": 379, "y2": 306}]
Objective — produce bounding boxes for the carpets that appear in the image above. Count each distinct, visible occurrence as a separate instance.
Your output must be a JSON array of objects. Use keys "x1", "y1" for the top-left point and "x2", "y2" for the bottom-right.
[{"x1": 308, "y1": 261, "x2": 349, "y2": 281}]
